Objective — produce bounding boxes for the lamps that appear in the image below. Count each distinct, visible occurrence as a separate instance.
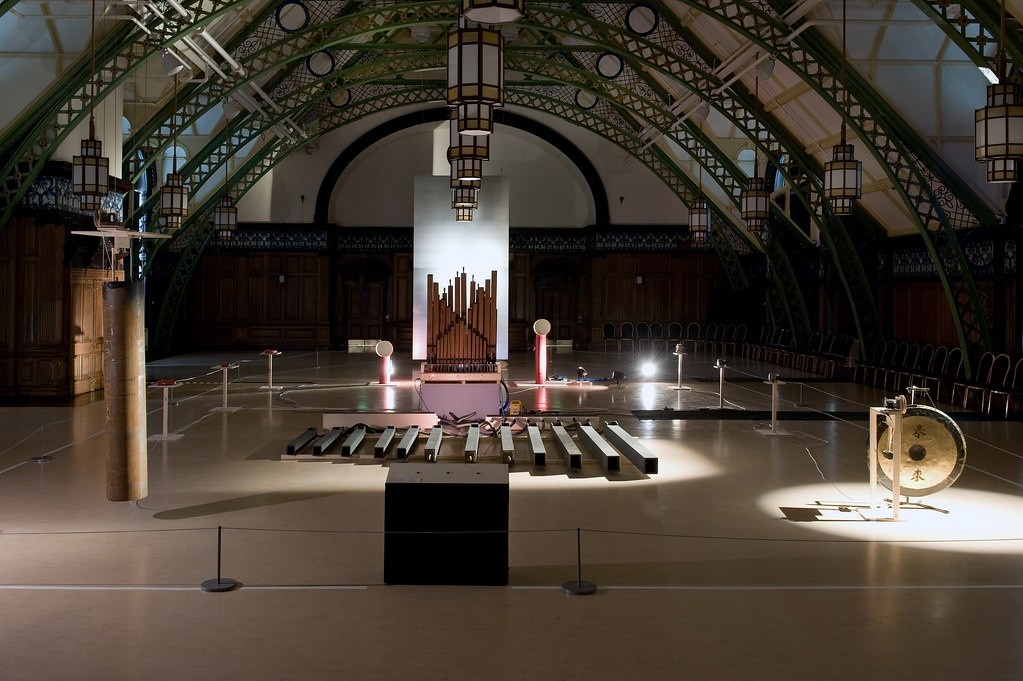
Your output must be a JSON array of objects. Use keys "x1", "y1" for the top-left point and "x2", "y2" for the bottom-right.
[
  {"x1": 412, "y1": 25, "x2": 430, "y2": 43},
  {"x1": 741, "y1": 48, "x2": 770, "y2": 232},
  {"x1": 449, "y1": 107, "x2": 490, "y2": 182},
  {"x1": 691, "y1": 100, "x2": 713, "y2": 123},
  {"x1": 160, "y1": 48, "x2": 183, "y2": 76},
  {"x1": 611, "y1": 371, "x2": 624, "y2": 385},
  {"x1": 447, "y1": 146, "x2": 482, "y2": 206},
  {"x1": 459, "y1": 0, "x2": 525, "y2": 23},
  {"x1": 576, "y1": 367, "x2": 588, "y2": 380},
  {"x1": 822, "y1": 0, "x2": 862, "y2": 217},
  {"x1": 159, "y1": 40, "x2": 188, "y2": 230},
  {"x1": 688, "y1": 117, "x2": 710, "y2": 244},
  {"x1": 217, "y1": 95, "x2": 240, "y2": 120},
  {"x1": 447, "y1": 14, "x2": 506, "y2": 136},
  {"x1": 451, "y1": 188, "x2": 478, "y2": 222},
  {"x1": 215, "y1": 92, "x2": 237, "y2": 241},
  {"x1": 975, "y1": 0, "x2": 1022, "y2": 184},
  {"x1": 754, "y1": 50, "x2": 778, "y2": 79},
  {"x1": 73, "y1": 1, "x2": 110, "y2": 213}
]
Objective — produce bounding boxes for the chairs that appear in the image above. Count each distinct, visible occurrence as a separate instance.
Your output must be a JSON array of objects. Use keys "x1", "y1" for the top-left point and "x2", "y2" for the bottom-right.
[
  {"x1": 667, "y1": 322, "x2": 962, "y2": 419},
  {"x1": 987, "y1": 358, "x2": 1023, "y2": 417},
  {"x1": 951, "y1": 352, "x2": 997, "y2": 407},
  {"x1": 602, "y1": 321, "x2": 620, "y2": 351},
  {"x1": 619, "y1": 322, "x2": 635, "y2": 351},
  {"x1": 965, "y1": 354, "x2": 1012, "y2": 413},
  {"x1": 636, "y1": 322, "x2": 651, "y2": 351},
  {"x1": 650, "y1": 322, "x2": 666, "y2": 351}
]
